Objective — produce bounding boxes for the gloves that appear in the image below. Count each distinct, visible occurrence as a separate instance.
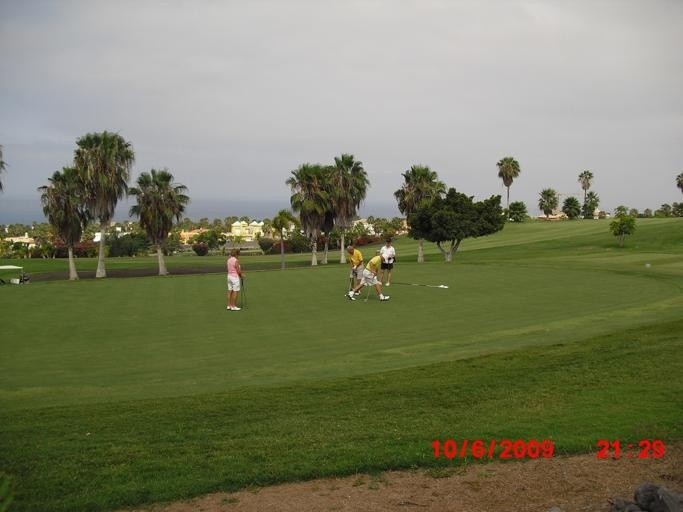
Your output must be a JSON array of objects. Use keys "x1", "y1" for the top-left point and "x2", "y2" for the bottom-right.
[{"x1": 352, "y1": 265, "x2": 356, "y2": 270}]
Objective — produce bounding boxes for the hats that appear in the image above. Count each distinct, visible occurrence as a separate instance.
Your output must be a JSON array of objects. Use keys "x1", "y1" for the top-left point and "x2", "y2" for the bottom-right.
[{"x1": 383, "y1": 255, "x2": 388, "y2": 264}]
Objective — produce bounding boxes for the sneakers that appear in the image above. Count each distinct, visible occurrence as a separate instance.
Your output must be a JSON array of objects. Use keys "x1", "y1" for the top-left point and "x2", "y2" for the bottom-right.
[
  {"x1": 380, "y1": 295, "x2": 390, "y2": 300},
  {"x1": 347, "y1": 289, "x2": 361, "y2": 301},
  {"x1": 226, "y1": 306, "x2": 241, "y2": 311},
  {"x1": 385, "y1": 283, "x2": 390, "y2": 287}
]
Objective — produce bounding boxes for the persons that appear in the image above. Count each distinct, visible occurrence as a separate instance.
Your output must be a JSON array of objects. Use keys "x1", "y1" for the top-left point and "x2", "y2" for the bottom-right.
[
  {"x1": 379, "y1": 237, "x2": 396, "y2": 286},
  {"x1": 347, "y1": 254, "x2": 390, "y2": 301},
  {"x1": 346, "y1": 245, "x2": 365, "y2": 296},
  {"x1": 226, "y1": 248, "x2": 246, "y2": 311}
]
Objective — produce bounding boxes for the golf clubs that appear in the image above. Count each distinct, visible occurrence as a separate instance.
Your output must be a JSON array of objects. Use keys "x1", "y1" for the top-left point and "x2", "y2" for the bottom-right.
[
  {"x1": 345, "y1": 267, "x2": 354, "y2": 296},
  {"x1": 239, "y1": 276, "x2": 247, "y2": 309},
  {"x1": 386, "y1": 256, "x2": 390, "y2": 285},
  {"x1": 364, "y1": 273, "x2": 375, "y2": 303}
]
